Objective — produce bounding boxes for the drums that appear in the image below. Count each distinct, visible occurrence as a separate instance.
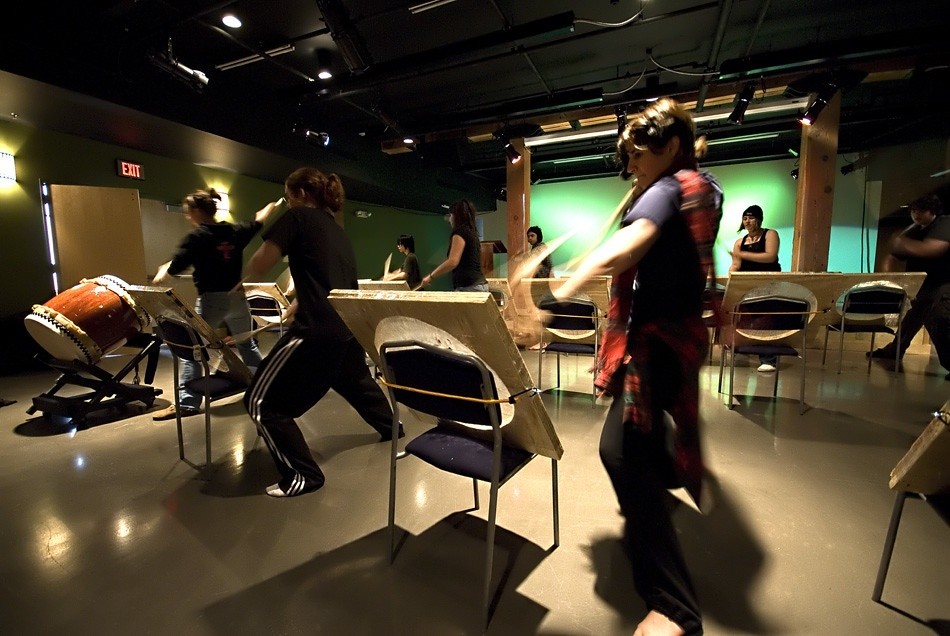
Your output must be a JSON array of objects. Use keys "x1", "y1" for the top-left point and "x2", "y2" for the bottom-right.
[{"x1": 24, "y1": 273, "x2": 154, "y2": 366}]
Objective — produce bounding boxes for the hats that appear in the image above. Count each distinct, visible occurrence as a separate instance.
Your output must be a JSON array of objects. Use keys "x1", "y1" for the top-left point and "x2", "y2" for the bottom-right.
[{"x1": 744, "y1": 206, "x2": 763, "y2": 220}]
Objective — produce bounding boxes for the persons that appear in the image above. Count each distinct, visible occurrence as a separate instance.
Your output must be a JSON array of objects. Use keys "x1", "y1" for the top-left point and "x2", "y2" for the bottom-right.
[
  {"x1": 380, "y1": 234, "x2": 424, "y2": 291},
  {"x1": 242, "y1": 168, "x2": 405, "y2": 498},
  {"x1": 153, "y1": 188, "x2": 280, "y2": 421},
  {"x1": 536, "y1": 98, "x2": 723, "y2": 635},
  {"x1": 527, "y1": 226, "x2": 552, "y2": 278},
  {"x1": 421, "y1": 198, "x2": 489, "y2": 293},
  {"x1": 730, "y1": 205, "x2": 782, "y2": 372},
  {"x1": 866, "y1": 194, "x2": 950, "y2": 382}
]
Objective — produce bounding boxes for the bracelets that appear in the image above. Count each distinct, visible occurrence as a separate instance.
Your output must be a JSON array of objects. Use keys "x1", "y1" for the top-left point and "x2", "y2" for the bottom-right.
[{"x1": 430, "y1": 272, "x2": 433, "y2": 280}]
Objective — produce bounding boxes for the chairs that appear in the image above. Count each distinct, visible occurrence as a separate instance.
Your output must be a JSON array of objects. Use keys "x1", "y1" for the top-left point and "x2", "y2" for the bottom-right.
[
  {"x1": 539, "y1": 296, "x2": 605, "y2": 409},
  {"x1": 717, "y1": 295, "x2": 811, "y2": 411},
  {"x1": 248, "y1": 295, "x2": 283, "y2": 340},
  {"x1": 381, "y1": 340, "x2": 560, "y2": 632},
  {"x1": 159, "y1": 318, "x2": 261, "y2": 470},
  {"x1": 823, "y1": 287, "x2": 905, "y2": 378},
  {"x1": 702, "y1": 289, "x2": 728, "y2": 367}
]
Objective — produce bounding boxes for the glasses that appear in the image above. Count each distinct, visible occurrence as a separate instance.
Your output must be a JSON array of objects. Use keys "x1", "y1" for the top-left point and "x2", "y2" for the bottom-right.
[{"x1": 911, "y1": 209, "x2": 927, "y2": 214}]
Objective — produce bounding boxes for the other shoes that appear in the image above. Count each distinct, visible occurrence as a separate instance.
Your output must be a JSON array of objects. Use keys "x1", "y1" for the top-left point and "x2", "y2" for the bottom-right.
[
  {"x1": 867, "y1": 349, "x2": 900, "y2": 363},
  {"x1": 154, "y1": 404, "x2": 195, "y2": 419},
  {"x1": 758, "y1": 364, "x2": 777, "y2": 372},
  {"x1": 529, "y1": 343, "x2": 547, "y2": 349},
  {"x1": 266, "y1": 483, "x2": 289, "y2": 497}
]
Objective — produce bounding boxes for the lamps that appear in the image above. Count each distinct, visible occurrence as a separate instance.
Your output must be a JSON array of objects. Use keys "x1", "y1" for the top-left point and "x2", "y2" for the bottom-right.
[
  {"x1": 840, "y1": 153, "x2": 871, "y2": 176},
  {"x1": 378, "y1": 106, "x2": 417, "y2": 147},
  {"x1": 148, "y1": 45, "x2": 209, "y2": 95},
  {"x1": 293, "y1": 123, "x2": 330, "y2": 149},
  {"x1": 799, "y1": 82, "x2": 838, "y2": 126},
  {"x1": 791, "y1": 168, "x2": 800, "y2": 180},
  {"x1": 725, "y1": 82, "x2": 758, "y2": 125},
  {"x1": 315, "y1": 50, "x2": 332, "y2": 80},
  {"x1": 495, "y1": 132, "x2": 521, "y2": 165}
]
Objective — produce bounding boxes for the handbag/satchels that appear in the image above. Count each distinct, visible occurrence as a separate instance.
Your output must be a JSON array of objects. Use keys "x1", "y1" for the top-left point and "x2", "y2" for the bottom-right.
[{"x1": 931, "y1": 284, "x2": 950, "y2": 321}]
